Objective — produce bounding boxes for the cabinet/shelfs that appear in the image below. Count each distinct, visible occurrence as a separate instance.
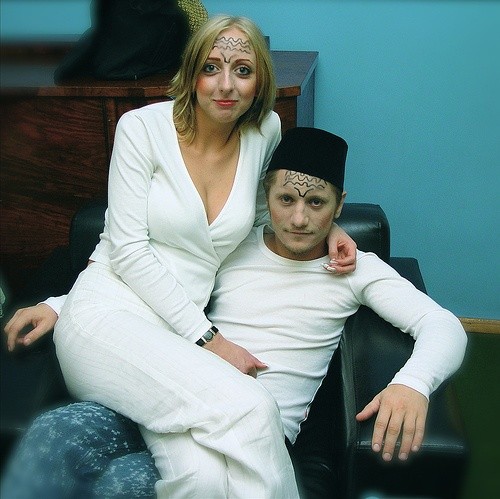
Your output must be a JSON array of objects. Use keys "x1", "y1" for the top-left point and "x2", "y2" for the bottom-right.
[{"x1": 1, "y1": 40, "x2": 320, "y2": 292}]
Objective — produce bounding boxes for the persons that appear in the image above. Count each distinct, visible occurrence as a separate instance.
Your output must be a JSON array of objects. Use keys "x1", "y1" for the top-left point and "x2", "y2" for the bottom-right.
[
  {"x1": 0, "y1": 127, "x2": 468, "y2": 498},
  {"x1": 53, "y1": 15, "x2": 358, "y2": 498}
]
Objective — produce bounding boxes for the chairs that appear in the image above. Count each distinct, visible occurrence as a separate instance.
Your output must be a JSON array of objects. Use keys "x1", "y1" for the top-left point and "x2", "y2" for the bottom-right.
[{"x1": 1, "y1": 202, "x2": 467, "y2": 499}]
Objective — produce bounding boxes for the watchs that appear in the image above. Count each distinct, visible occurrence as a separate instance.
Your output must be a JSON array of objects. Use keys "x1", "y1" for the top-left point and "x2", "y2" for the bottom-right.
[{"x1": 196, "y1": 326, "x2": 219, "y2": 347}]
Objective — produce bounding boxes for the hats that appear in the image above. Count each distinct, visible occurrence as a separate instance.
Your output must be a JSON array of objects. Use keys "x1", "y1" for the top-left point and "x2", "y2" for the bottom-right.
[{"x1": 266, "y1": 127, "x2": 349, "y2": 192}]
